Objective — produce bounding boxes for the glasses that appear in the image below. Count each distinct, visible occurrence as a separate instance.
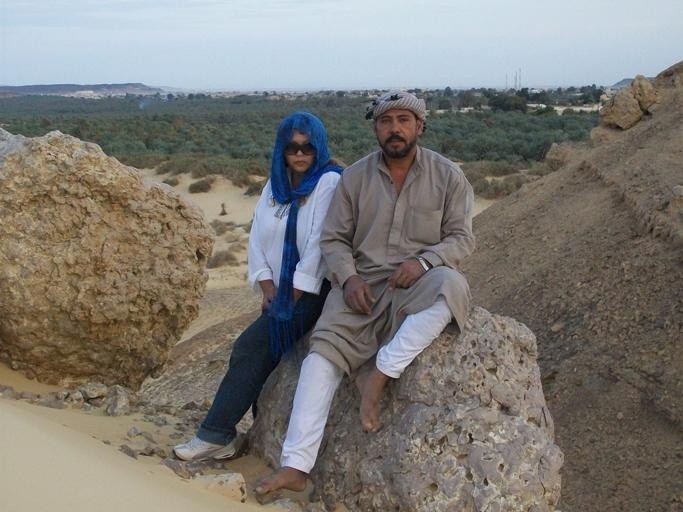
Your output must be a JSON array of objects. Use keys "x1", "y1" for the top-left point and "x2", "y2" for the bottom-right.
[{"x1": 284, "y1": 142, "x2": 314, "y2": 156}]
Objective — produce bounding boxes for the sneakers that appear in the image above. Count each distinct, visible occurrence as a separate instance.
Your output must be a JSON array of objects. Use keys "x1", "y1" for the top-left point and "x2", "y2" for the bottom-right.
[{"x1": 173, "y1": 435, "x2": 237, "y2": 463}]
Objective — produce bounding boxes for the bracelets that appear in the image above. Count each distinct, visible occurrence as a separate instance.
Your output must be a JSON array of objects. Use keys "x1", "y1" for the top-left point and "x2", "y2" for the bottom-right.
[{"x1": 417, "y1": 256, "x2": 429, "y2": 272}]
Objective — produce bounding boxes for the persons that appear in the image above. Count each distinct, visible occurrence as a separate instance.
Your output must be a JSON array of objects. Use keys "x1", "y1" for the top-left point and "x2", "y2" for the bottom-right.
[
  {"x1": 171, "y1": 111, "x2": 347, "y2": 463},
  {"x1": 250, "y1": 92, "x2": 476, "y2": 499}
]
